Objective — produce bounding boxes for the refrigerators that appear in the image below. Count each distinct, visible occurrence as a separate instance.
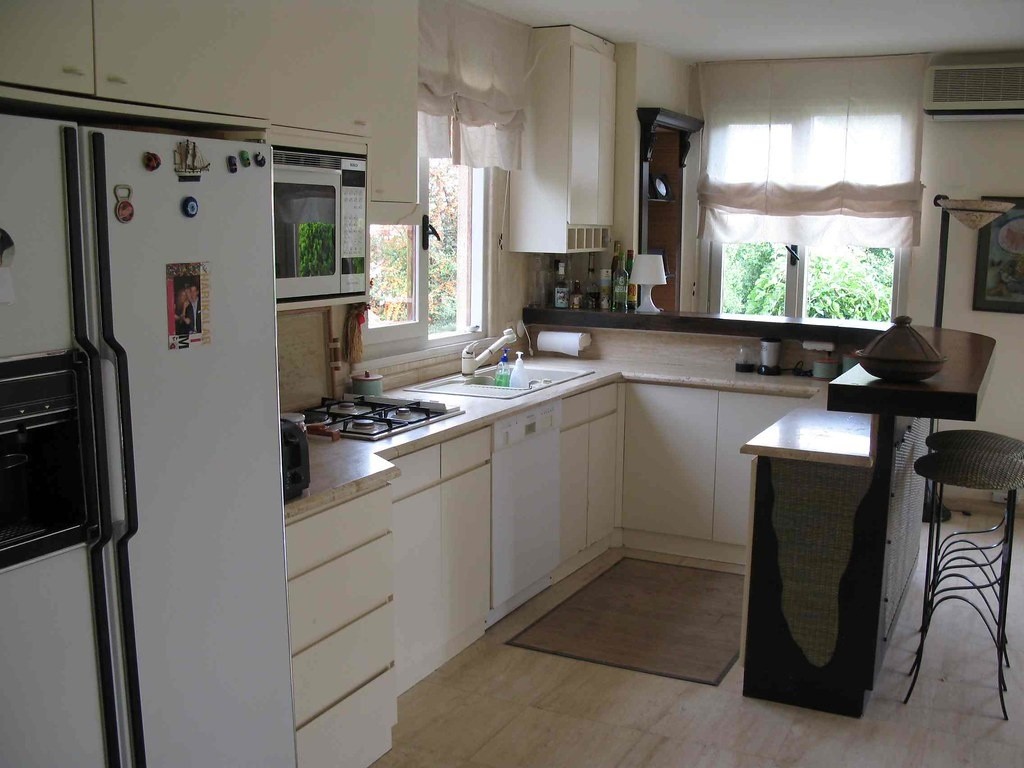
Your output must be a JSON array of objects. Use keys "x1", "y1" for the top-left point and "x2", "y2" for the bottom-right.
[{"x1": 0, "y1": 112, "x2": 297, "y2": 768}]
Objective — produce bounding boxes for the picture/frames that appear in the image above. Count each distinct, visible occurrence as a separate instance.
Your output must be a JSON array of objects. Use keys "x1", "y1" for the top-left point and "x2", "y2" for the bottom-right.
[{"x1": 973, "y1": 196, "x2": 1024, "y2": 314}]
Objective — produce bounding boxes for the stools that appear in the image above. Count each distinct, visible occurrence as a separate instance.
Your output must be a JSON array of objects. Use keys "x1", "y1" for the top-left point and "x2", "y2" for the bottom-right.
[{"x1": 902, "y1": 431, "x2": 1024, "y2": 720}]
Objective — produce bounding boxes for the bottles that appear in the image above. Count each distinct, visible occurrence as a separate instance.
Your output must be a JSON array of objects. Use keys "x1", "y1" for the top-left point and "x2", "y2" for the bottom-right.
[
  {"x1": 532, "y1": 258, "x2": 546, "y2": 310},
  {"x1": 554, "y1": 263, "x2": 570, "y2": 309},
  {"x1": 612, "y1": 241, "x2": 621, "y2": 312},
  {"x1": 571, "y1": 280, "x2": 583, "y2": 310},
  {"x1": 625, "y1": 250, "x2": 638, "y2": 311},
  {"x1": 584, "y1": 252, "x2": 599, "y2": 312},
  {"x1": 613, "y1": 251, "x2": 629, "y2": 313}
]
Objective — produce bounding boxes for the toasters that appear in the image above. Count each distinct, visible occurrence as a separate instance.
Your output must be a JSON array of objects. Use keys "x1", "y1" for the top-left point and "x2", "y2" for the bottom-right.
[{"x1": 280, "y1": 412, "x2": 310, "y2": 503}]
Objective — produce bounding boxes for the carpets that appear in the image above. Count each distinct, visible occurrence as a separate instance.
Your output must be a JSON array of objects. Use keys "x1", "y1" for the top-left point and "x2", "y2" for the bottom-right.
[{"x1": 503, "y1": 555, "x2": 739, "y2": 687}]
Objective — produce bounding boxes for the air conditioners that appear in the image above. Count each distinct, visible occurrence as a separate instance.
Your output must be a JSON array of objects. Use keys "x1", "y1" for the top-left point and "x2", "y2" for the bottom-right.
[{"x1": 920, "y1": 62, "x2": 1024, "y2": 121}]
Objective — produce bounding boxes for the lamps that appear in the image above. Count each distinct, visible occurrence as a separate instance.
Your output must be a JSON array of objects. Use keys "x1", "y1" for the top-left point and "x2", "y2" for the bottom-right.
[{"x1": 630, "y1": 254, "x2": 667, "y2": 311}]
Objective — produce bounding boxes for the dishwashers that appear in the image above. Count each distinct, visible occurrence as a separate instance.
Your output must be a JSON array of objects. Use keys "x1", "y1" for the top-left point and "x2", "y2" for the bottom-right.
[{"x1": 490, "y1": 399, "x2": 560, "y2": 611}]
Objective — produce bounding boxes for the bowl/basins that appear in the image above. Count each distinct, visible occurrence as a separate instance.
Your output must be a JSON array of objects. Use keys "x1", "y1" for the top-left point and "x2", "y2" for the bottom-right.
[{"x1": 854, "y1": 349, "x2": 949, "y2": 382}]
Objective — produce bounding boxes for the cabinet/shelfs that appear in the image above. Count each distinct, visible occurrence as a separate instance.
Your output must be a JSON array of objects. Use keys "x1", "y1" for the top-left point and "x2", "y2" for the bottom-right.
[
  {"x1": 512, "y1": 23, "x2": 617, "y2": 253},
  {"x1": 284, "y1": 489, "x2": 401, "y2": 768},
  {"x1": 388, "y1": 429, "x2": 492, "y2": 698},
  {"x1": 274, "y1": 0, "x2": 422, "y2": 202},
  {"x1": 555, "y1": 383, "x2": 628, "y2": 572},
  {"x1": 0, "y1": 1, "x2": 274, "y2": 121},
  {"x1": 618, "y1": 384, "x2": 807, "y2": 547}
]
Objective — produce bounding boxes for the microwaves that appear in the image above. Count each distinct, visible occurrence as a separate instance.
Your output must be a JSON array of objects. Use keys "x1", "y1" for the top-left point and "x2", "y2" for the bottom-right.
[{"x1": 271, "y1": 145, "x2": 368, "y2": 304}]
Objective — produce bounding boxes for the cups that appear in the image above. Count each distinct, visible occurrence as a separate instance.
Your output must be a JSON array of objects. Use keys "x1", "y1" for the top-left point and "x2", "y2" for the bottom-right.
[{"x1": 351, "y1": 370, "x2": 383, "y2": 396}]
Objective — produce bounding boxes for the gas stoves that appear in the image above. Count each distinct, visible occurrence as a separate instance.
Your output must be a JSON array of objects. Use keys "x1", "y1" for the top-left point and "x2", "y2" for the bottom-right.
[{"x1": 293, "y1": 396, "x2": 466, "y2": 441}]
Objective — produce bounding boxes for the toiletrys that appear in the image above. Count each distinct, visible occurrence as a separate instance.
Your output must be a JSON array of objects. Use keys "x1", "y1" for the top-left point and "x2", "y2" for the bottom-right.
[
  {"x1": 494, "y1": 347, "x2": 511, "y2": 387},
  {"x1": 509, "y1": 351, "x2": 529, "y2": 388}
]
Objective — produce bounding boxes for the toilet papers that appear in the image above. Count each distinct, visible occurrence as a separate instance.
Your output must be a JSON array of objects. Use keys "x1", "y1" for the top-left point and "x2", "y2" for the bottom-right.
[{"x1": 536, "y1": 330, "x2": 592, "y2": 357}]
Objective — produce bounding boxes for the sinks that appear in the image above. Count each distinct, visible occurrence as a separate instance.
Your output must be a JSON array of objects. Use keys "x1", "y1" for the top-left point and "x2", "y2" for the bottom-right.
[{"x1": 402, "y1": 364, "x2": 594, "y2": 398}]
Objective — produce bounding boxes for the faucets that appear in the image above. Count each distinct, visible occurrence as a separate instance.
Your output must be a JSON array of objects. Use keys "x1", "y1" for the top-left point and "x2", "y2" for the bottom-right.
[{"x1": 461, "y1": 328, "x2": 517, "y2": 377}]
